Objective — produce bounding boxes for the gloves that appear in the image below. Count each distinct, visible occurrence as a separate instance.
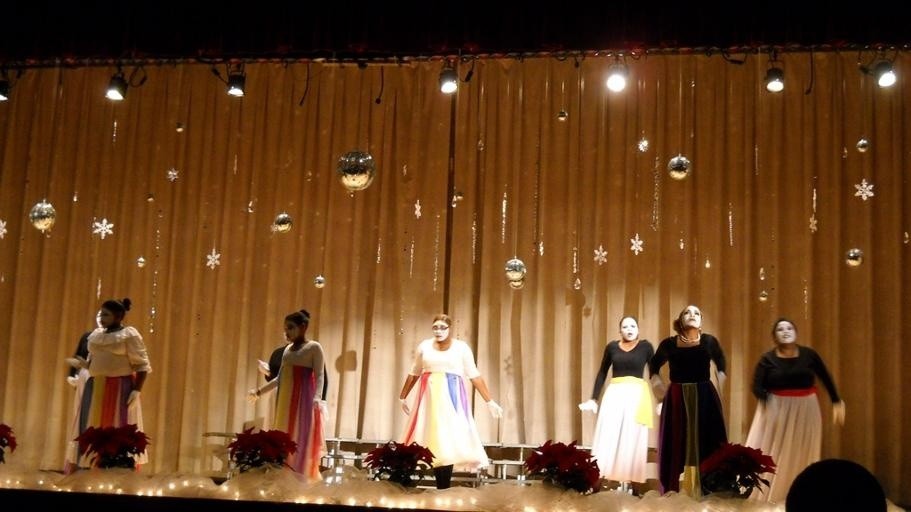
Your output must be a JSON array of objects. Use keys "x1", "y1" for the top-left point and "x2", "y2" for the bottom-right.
[
  {"x1": 259, "y1": 362, "x2": 272, "y2": 377},
  {"x1": 486, "y1": 398, "x2": 504, "y2": 419},
  {"x1": 578, "y1": 399, "x2": 599, "y2": 415},
  {"x1": 398, "y1": 397, "x2": 412, "y2": 417},
  {"x1": 312, "y1": 396, "x2": 330, "y2": 422},
  {"x1": 67, "y1": 374, "x2": 79, "y2": 387},
  {"x1": 127, "y1": 389, "x2": 142, "y2": 411},
  {"x1": 247, "y1": 389, "x2": 260, "y2": 406},
  {"x1": 832, "y1": 399, "x2": 846, "y2": 427}
]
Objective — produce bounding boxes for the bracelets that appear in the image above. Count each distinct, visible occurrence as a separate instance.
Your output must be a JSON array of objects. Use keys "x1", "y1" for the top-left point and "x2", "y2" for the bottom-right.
[
  {"x1": 399, "y1": 397, "x2": 408, "y2": 405},
  {"x1": 257, "y1": 387, "x2": 264, "y2": 395}
]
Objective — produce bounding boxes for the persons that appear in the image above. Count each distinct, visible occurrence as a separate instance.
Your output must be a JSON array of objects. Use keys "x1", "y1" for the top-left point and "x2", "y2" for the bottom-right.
[
  {"x1": 247, "y1": 308, "x2": 325, "y2": 485},
  {"x1": 68, "y1": 297, "x2": 152, "y2": 470},
  {"x1": 649, "y1": 304, "x2": 730, "y2": 496},
  {"x1": 577, "y1": 315, "x2": 666, "y2": 493},
  {"x1": 258, "y1": 330, "x2": 328, "y2": 399},
  {"x1": 737, "y1": 318, "x2": 849, "y2": 504},
  {"x1": 395, "y1": 313, "x2": 510, "y2": 489},
  {"x1": 66, "y1": 310, "x2": 103, "y2": 477}
]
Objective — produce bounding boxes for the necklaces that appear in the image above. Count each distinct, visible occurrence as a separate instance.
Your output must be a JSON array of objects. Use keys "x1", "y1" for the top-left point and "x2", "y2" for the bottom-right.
[{"x1": 677, "y1": 332, "x2": 703, "y2": 344}]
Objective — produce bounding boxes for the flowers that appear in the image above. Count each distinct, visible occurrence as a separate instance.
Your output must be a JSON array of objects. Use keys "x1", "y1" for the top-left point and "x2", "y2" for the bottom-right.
[
  {"x1": 71, "y1": 424, "x2": 152, "y2": 471},
  {"x1": 360, "y1": 440, "x2": 437, "y2": 487},
  {"x1": 0, "y1": 424, "x2": 18, "y2": 464},
  {"x1": 698, "y1": 442, "x2": 778, "y2": 499},
  {"x1": 519, "y1": 438, "x2": 601, "y2": 495},
  {"x1": 225, "y1": 427, "x2": 300, "y2": 473}
]
